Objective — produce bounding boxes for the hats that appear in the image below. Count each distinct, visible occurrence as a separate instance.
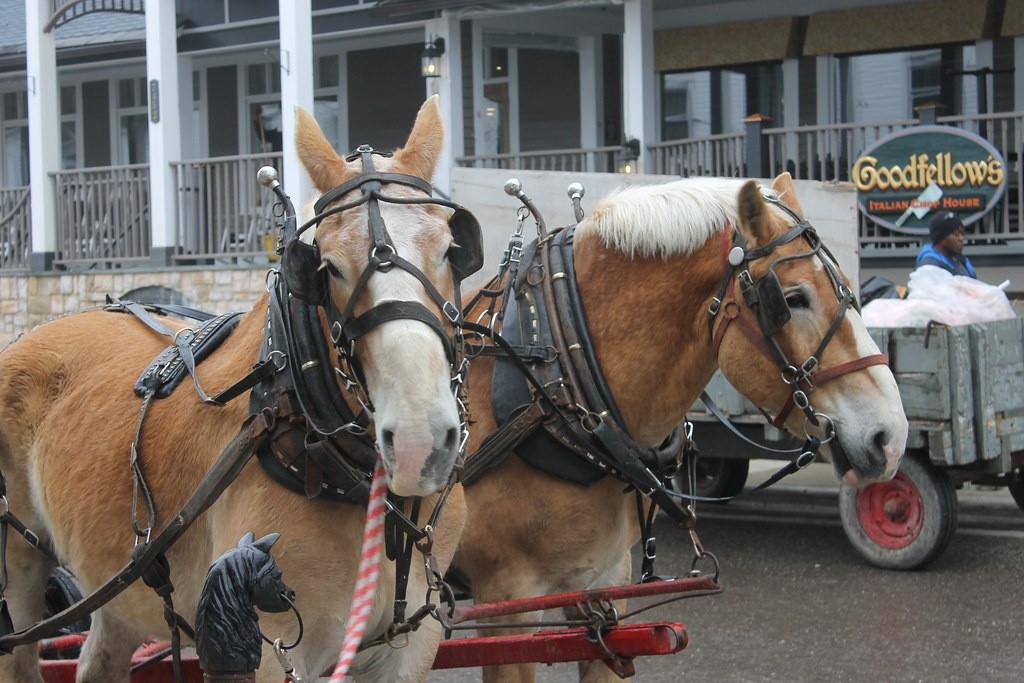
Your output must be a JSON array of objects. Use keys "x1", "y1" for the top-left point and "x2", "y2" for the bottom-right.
[{"x1": 929, "y1": 217, "x2": 963, "y2": 246}]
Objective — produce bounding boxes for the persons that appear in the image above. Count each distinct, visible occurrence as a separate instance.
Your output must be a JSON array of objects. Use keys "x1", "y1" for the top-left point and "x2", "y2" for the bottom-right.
[{"x1": 916, "y1": 210, "x2": 976, "y2": 279}]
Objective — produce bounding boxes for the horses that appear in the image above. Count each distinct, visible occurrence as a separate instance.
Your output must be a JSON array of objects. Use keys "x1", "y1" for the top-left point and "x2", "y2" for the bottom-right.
[
  {"x1": 0, "y1": 93, "x2": 467, "y2": 683},
  {"x1": 195, "y1": 532, "x2": 295, "y2": 672},
  {"x1": 453, "y1": 172, "x2": 909, "y2": 683}
]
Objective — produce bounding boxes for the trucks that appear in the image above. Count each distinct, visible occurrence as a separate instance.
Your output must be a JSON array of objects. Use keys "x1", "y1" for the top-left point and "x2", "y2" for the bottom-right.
[{"x1": 670, "y1": 261, "x2": 1024, "y2": 569}]
